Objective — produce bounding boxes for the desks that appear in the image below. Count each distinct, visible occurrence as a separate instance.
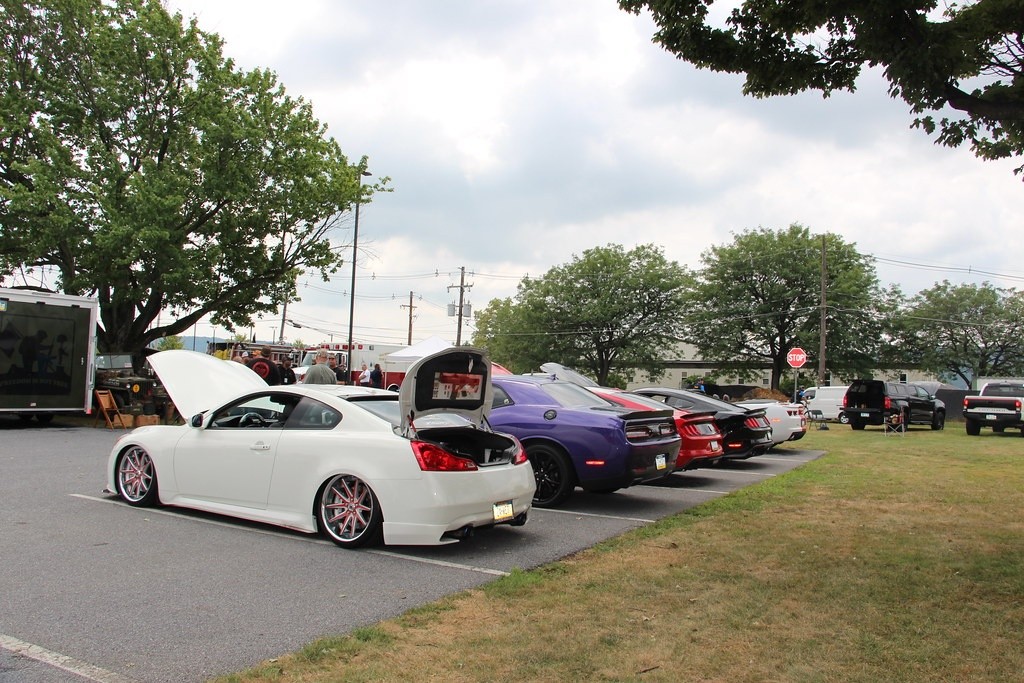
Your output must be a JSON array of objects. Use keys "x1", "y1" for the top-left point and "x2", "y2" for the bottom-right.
[{"x1": 154, "y1": 396, "x2": 168, "y2": 413}]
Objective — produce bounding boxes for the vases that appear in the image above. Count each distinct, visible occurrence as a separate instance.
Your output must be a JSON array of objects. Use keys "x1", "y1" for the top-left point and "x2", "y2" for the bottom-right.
[{"x1": 163, "y1": 401, "x2": 175, "y2": 419}]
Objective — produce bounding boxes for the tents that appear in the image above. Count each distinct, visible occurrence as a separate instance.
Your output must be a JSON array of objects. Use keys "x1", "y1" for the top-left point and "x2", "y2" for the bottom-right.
[{"x1": 384, "y1": 335, "x2": 455, "y2": 390}]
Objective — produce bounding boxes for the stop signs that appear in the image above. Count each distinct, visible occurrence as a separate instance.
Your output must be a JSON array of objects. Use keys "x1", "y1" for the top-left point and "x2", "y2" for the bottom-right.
[{"x1": 786, "y1": 348, "x2": 807, "y2": 368}]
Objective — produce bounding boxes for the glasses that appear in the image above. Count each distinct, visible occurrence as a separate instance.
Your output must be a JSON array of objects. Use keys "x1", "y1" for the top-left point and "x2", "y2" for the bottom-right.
[{"x1": 329, "y1": 358, "x2": 335, "y2": 360}]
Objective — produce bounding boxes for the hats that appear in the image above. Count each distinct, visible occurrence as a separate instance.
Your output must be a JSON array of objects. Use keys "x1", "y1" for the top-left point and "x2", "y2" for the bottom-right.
[{"x1": 261, "y1": 347, "x2": 271, "y2": 356}]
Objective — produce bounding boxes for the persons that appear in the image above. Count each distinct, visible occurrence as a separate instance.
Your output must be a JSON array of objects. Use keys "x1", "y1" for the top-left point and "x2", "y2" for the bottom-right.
[
  {"x1": 234, "y1": 350, "x2": 260, "y2": 366},
  {"x1": 370, "y1": 364, "x2": 383, "y2": 388},
  {"x1": 328, "y1": 355, "x2": 347, "y2": 384},
  {"x1": 693, "y1": 377, "x2": 705, "y2": 392},
  {"x1": 279, "y1": 357, "x2": 306, "y2": 385},
  {"x1": 246, "y1": 345, "x2": 281, "y2": 386},
  {"x1": 359, "y1": 364, "x2": 370, "y2": 386},
  {"x1": 304, "y1": 349, "x2": 336, "y2": 384}
]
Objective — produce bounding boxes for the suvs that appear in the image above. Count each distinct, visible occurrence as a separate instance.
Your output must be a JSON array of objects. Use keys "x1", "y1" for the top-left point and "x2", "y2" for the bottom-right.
[
  {"x1": 96, "y1": 354, "x2": 163, "y2": 415},
  {"x1": 842, "y1": 378, "x2": 946, "y2": 432}
]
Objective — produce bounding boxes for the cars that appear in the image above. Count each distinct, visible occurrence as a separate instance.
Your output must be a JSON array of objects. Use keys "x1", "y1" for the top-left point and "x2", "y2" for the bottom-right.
[
  {"x1": 586, "y1": 386, "x2": 726, "y2": 474},
  {"x1": 692, "y1": 385, "x2": 807, "y2": 449},
  {"x1": 103, "y1": 349, "x2": 537, "y2": 550},
  {"x1": 542, "y1": 363, "x2": 773, "y2": 466},
  {"x1": 488, "y1": 376, "x2": 681, "y2": 508}
]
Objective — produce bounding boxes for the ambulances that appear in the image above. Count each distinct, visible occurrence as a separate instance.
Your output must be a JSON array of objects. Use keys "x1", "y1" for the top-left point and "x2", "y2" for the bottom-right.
[{"x1": 290, "y1": 343, "x2": 409, "y2": 386}]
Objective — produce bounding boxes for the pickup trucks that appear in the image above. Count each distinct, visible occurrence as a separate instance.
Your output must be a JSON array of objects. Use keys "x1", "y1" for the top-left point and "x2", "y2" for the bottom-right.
[{"x1": 961, "y1": 381, "x2": 1024, "y2": 437}]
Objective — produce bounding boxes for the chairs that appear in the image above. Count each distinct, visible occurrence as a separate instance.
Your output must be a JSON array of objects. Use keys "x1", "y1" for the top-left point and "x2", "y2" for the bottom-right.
[
  {"x1": 94, "y1": 389, "x2": 127, "y2": 430},
  {"x1": 884, "y1": 406, "x2": 905, "y2": 438},
  {"x1": 270, "y1": 403, "x2": 295, "y2": 428},
  {"x1": 808, "y1": 410, "x2": 827, "y2": 431}
]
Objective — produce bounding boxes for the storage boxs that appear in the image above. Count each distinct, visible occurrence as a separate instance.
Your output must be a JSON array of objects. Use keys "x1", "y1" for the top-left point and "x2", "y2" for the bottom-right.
[
  {"x1": 152, "y1": 386, "x2": 164, "y2": 395},
  {"x1": 113, "y1": 400, "x2": 160, "y2": 429}
]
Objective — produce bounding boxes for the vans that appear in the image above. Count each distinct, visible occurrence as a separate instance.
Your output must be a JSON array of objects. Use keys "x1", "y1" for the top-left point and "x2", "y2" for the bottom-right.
[{"x1": 802, "y1": 386, "x2": 849, "y2": 424}]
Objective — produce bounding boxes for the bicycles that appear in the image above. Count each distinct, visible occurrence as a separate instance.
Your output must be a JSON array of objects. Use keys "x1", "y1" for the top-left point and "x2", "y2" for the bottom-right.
[{"x1": 803, "y1": 402, "x2": 812, "y2": 422}]
